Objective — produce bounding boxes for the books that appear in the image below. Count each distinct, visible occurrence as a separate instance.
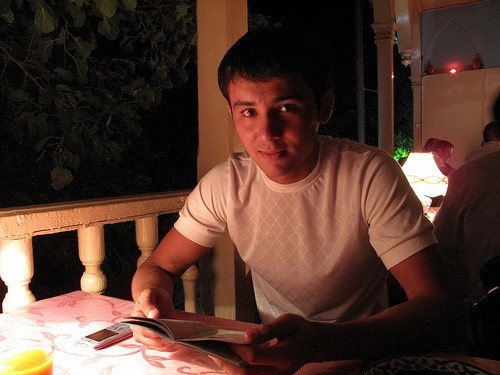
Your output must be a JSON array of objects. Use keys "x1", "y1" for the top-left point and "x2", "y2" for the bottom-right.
[{"x1": 122, "y1": 314, "x2": 272, "y2": 368}]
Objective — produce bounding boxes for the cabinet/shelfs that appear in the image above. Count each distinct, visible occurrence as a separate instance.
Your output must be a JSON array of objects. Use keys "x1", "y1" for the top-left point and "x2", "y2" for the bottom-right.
[{"x1": 422, "y1": 66, "x2": 500, "y2": 168}]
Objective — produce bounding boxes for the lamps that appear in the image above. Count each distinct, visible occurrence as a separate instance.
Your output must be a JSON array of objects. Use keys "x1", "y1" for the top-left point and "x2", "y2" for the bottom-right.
[{"x1": 401, "y1": 151, "x2": 448, "y2": 215}]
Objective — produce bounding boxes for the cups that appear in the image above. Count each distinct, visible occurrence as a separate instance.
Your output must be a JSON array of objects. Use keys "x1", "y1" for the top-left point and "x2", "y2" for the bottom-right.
[{"x1": 0, "y1": 335, "x2": 55, "y2": 375}]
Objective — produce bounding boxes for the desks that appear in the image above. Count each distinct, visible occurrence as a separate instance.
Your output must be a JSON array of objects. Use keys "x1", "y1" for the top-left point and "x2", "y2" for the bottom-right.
[{"x1": 0, "y1": 288, "x2": 500, "y2": 375}]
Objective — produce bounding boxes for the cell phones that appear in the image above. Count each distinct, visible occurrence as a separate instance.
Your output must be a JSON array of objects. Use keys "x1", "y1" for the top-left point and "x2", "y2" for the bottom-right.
[{"x1": 79, "y1": 322, "x2": 132, "y2": 349}]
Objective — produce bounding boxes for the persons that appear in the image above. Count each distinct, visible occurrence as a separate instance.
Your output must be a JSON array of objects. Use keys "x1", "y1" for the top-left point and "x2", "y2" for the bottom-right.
[
  {"x1": 130, "y1": 28, "x2": 466, "y2": 366},
  {"x1": 421, "y1": 121, "x2": 500, "y2": 305}
]
